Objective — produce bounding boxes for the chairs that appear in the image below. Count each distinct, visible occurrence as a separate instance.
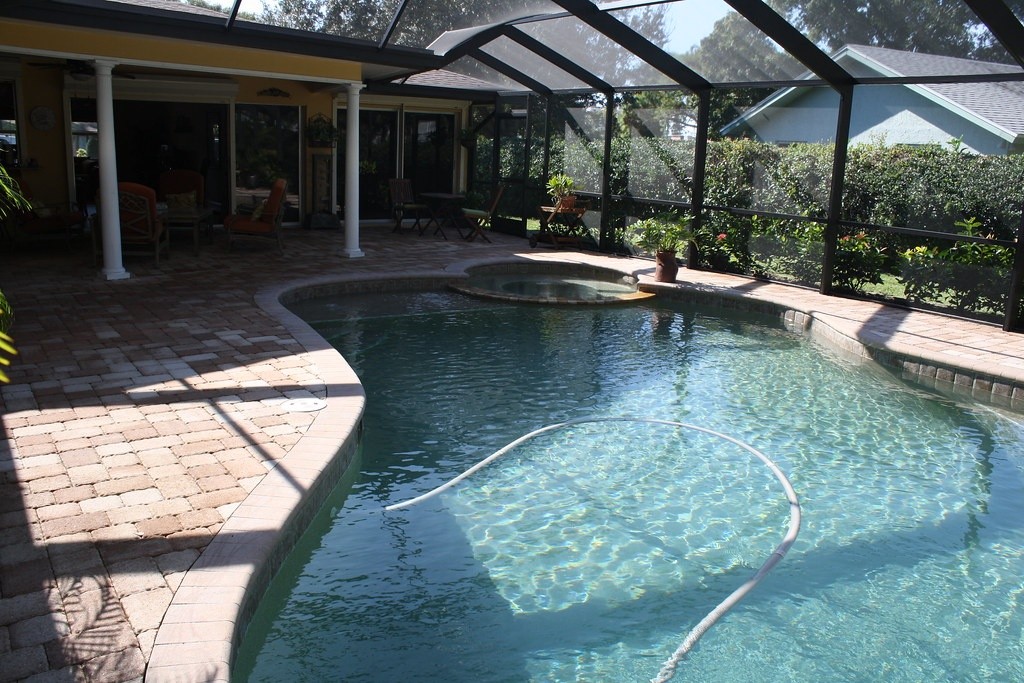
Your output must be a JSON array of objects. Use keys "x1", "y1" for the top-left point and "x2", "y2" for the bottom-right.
[
  {"x1": 389, "y1": 176, "x2": 432, "y2": 235},
  {"x1": 159, "y1": 169, "x2": 205, "y2": 209},
  {"x1": 11, "y1": 187, "x2": 86, "y2": 253},
  {"x1": 88, "y1": 182, "x2": 170, "y2": 267},
  {"x1": 224, "y1": 177, "x2": 287, "y2": 253}
]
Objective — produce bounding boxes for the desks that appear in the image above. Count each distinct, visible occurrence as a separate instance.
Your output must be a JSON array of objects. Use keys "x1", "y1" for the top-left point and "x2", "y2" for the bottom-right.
[
  {"x1": 527, "y1": 205, "x2": 588, "y2": 252},
  {"x1": 416, "y1": 193, "x2": 468, "y2": 240},
  {"x1": 161, "y1": 207, "x2": 214, "y2": 255}
]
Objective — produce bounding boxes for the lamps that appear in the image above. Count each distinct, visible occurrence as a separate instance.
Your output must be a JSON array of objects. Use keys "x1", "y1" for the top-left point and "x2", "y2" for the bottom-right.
[{"x1": 208, "y1": 120, "x2": 225, "y2": 145}]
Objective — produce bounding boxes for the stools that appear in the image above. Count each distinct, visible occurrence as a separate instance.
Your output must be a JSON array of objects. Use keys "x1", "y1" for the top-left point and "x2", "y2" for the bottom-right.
[{"x1": 464, "y1": 213, "x2": 494, "y2": 243}]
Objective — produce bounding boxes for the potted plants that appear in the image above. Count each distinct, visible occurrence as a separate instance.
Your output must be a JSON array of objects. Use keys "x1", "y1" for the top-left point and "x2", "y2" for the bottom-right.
[
  {"x1": 544, "y1": 172, "x2": 583, "y2": 208},
  {"x1": 302, "y1": 116, "x2": 336, "y2": 148},
  {"x1": 630, "y1": 205, "x2": 699, "y2": 283}
]
